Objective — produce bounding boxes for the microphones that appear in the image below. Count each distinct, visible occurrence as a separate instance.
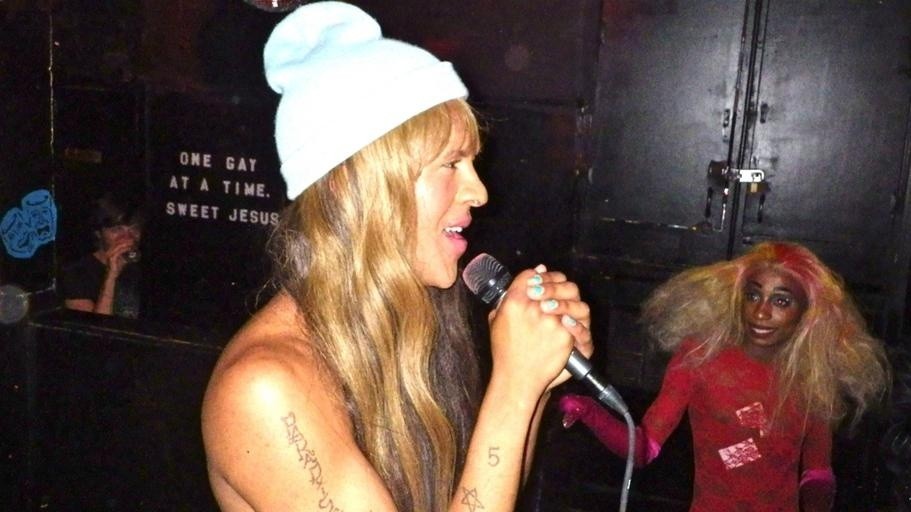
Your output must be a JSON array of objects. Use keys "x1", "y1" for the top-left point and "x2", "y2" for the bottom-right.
[{"x1": 462, "y1": 253, "x2": 630, "y2": 415}]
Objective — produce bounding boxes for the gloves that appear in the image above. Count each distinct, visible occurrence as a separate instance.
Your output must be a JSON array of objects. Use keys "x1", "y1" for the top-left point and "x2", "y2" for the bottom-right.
[{"x1": 551, "y1": 392, "x2": 660, "y2": 471}]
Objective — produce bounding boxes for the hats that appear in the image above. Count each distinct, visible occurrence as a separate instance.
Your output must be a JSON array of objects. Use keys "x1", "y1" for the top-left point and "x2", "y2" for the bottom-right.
[{"x1": 262, "y1": 2, "x2": 470, "y2": 201}]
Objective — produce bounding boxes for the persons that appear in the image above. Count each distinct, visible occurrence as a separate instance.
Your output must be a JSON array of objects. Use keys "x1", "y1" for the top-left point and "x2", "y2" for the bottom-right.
[
  {"x1": 199, "y1": 0, "x2": 595, "y2": 512},
  {"x1": 63, "y1": 183, "x2": 144, "y2": 322},
  {"x1": 560, "y1": 239, "x2": 894, "y2": 512}
]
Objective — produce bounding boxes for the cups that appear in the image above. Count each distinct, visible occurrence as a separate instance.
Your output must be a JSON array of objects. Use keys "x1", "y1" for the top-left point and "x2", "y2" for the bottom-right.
[{"x1": 117, "y1": 234, "x2": 141, "y2": 264}]
